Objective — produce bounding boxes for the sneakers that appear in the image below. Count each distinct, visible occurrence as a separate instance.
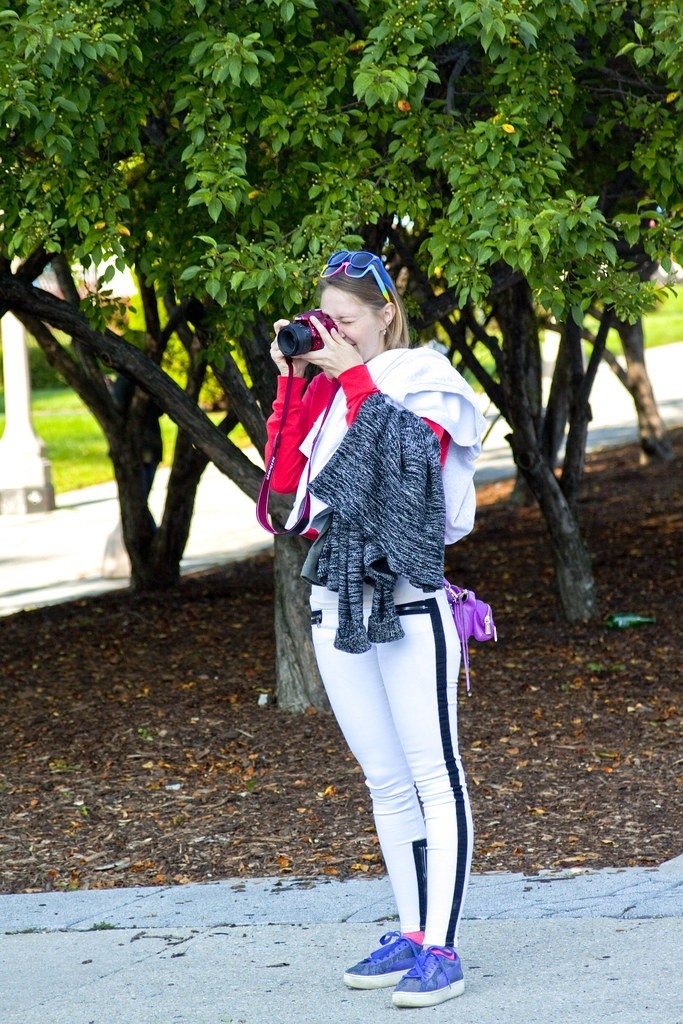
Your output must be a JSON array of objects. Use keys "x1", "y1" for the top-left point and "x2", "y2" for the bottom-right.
[
  {"x1": 393, "y1": 947, "x2": 466, "y2": 1007},
  {"x1": 342, "y1": 931, "x2": 423, "y2": 989}
]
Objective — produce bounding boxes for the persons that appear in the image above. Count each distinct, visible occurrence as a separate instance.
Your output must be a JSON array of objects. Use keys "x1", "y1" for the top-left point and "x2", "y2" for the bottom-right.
[{"x1": 264, "y1": 251, "x2": 483, "y2": 1007}]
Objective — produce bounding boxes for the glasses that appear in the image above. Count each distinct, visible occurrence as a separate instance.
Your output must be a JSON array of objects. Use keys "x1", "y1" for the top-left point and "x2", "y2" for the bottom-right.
[
  {"x1": 328, "y1": 250, "x2": 392, "y2": 289},
  {"x1": 321, "y1": 261, "x2": 392, "y2": 304}
]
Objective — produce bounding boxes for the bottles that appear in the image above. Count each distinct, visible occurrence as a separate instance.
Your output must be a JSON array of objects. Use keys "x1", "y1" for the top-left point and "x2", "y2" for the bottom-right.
[{"x1": 604, "y1": 612, "x2": 657, "y2": 629}]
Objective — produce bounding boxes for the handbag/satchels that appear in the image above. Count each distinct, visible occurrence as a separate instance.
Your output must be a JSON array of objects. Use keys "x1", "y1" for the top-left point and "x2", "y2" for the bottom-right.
[{"x1": 445, "y1": 585, "x2": 496, "y2": 643}]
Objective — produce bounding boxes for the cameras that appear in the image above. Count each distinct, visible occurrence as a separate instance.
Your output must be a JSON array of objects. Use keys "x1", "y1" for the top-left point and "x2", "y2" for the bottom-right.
[{"x1": 277, "y1": 308, "x2": 337, "y2": 357}]
